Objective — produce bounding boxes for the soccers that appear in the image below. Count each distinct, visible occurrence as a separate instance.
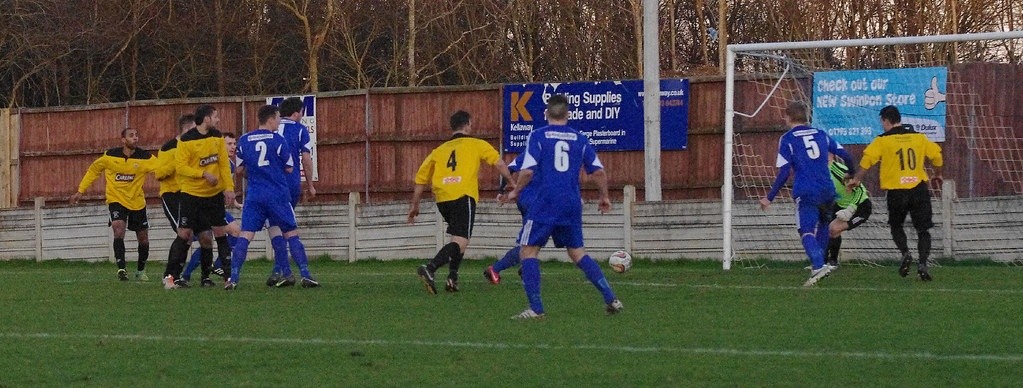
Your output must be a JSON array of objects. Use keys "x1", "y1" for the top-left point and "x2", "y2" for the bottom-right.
[{"x1": 609, "y1": 250, "x2": 633, "y2": 273}]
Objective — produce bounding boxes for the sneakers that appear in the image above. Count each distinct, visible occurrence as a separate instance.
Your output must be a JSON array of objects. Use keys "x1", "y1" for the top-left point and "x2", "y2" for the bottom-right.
[
  {"x1": 482, "y1": 265, "x2": 502, "y2": 285},
  {"x1": 265, "y1": 274, "x2": 296, "y2": 288},
  {"x1": 224, "y1": 280, "x2": 238, "y2": 291},
  {"x1": 135, "y1": 270, "x2": 149, "y2": 280},
  {"x1": 299, "y1": 276, "x2": 323, "y2": 288},
  {"x1": 445, "y1": 279, "x2": 460, "y2": 292},
  {"x1": 211, "y1": 262, "x2": 227, "y2": 278},
  {"x1": 173, "y1": 277, "x2": 190, "y2": 286},
  {"x1": 161, "y1": 273, "x2": 176, "y2": 290},
  {"x1": 116, "y1": 268, "x2": 129, "y2": 281},
  {"x1": 417, "y1": 264, "x2": 438, "y2": 296},
  {"x1": 199, "y1": 278, "x2": 216, "y2": 287},
  {"x1": 898, "y1": 252, "x2": 913, "y2": 277},
  {"x1": 917, "y1": 264, "x2": 933, "y2": 282},
  {"x1": 603, "y1": 299, "x2": 624, "y2": 316},
  {"x1": 803, "y1": 265, "x2": 832, "y2": 287},
  {"x1": 508, "y1": 307, "x2": 547, "y2": 320}
]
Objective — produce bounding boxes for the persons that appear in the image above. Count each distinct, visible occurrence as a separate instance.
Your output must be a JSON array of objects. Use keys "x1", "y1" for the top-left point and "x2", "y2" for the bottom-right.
[
  {"x1": 761, "y1": 101, "x2": 855, "y2": 285},
  {"x1": 175, "y1": 106, "x2": 235, "y2": 288},
  {"x1": 859, "y1": 104, "x2": 943, "y2": 281},
  {"x1": 409, "y1": 110, "x2": 518, "y2": 294},
  {"x1": 483, "y1": 150, "x2": 525, "y2": 284},
  {"x1": 231, "y1": 105, "x2": 320, "y2": 287},
  {"x1": 265, "y1": 98, "x2": 316, "y2": 287},
  {"x1": 825, "y1": 152, "x2": 873, "y2": 268},
  {"x1": 74, "y1": 128, "x2": 156, "y2": 281},
  {"x1": 508, "y1": 95, "x2": 622, "y2": 318},
  {"x1": 156, "y1": 113, "x2": 197, "y2": 287},
  {"x1": 181, "y1": 133, "x2": 239, "y2": 288}
]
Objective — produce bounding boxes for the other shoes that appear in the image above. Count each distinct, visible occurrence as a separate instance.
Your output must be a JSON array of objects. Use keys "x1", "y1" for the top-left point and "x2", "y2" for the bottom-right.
[
  {"x1": 804, "y1": 263, "x2": 819, "y2": 270},
  {"x1": 821, "y1": 263, "x2": 841, "y2": 271}
]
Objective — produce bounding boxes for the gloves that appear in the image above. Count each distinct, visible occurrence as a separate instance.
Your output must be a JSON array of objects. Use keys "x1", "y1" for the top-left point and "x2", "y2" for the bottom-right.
[{"x1": 835, "y1": 206, "x2": 856, "y2": 223}]
